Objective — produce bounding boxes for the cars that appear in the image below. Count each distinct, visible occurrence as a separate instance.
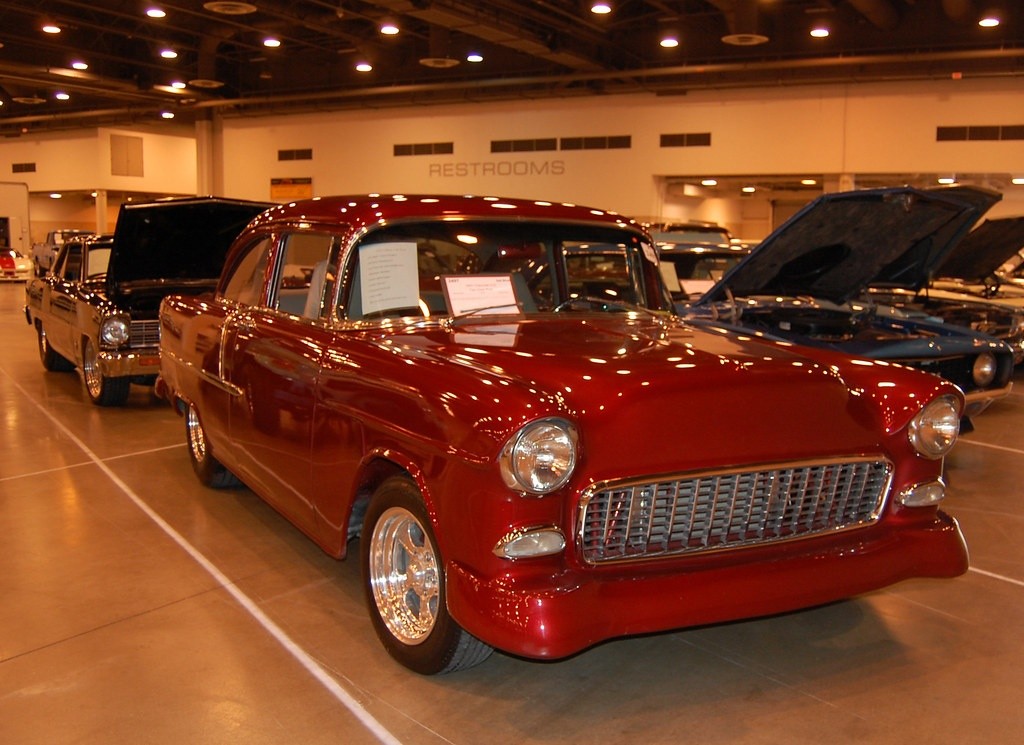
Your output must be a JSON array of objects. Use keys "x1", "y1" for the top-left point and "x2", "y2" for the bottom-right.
[
  {"x1": 491, "y1": 181, "x2": 1023, "y2": 439},
  {"x1": 160, "y1": 191, "x2": 969, "y2": 677},
  {"x1": 25, "y1": 196, "x2": 281, "y2": 412},
  {"x1": 0, "y1": 247, "x2": 34, "y2": 281}
]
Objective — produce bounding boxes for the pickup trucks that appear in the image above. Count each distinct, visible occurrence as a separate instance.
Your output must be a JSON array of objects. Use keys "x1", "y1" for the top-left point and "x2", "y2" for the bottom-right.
[{"x1": 32, "y1": 230, "x2": 93, "y2": 277}]
